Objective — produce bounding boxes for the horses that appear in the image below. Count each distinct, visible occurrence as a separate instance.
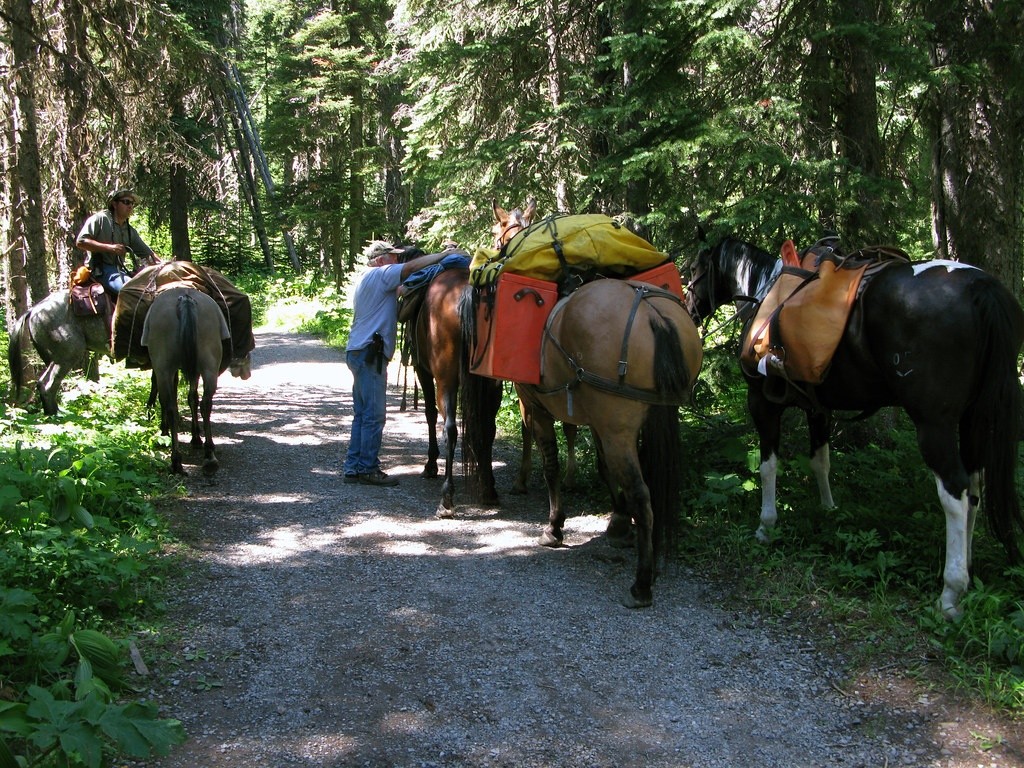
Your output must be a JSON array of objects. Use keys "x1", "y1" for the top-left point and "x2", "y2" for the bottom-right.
[
  {"x1": 9, "y1": 272, "x2": 178, "y2": 415},
  {"x1": 686, "y1": 229, "x2": 1024, "y2": 624},
  {"x1": 147, "y1": 288, "x2": 222, "y2": 479},
  {"x1": 378, "y1": 198, "x2": 702, "y2": 608}
]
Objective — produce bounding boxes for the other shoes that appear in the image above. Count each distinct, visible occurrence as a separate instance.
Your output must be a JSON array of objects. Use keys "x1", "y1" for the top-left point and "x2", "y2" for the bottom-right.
[
  {"x1": 344, "y1": 474, "x2": 359, "y2": 483},
  {"x1": 359, "y1": 471, "x2": 399, "y2": 486}
]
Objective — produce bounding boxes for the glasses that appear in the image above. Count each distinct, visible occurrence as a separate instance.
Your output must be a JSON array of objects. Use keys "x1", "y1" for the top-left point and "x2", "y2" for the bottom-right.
[{"x1": 120, "y1": 200, "x2": 134, "y2": 206}]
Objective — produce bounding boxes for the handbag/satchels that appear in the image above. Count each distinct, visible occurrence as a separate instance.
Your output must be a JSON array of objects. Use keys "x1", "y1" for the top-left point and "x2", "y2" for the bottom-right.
[{"x1": 71, "y1": 282, "x2": 106, "y2": 318}]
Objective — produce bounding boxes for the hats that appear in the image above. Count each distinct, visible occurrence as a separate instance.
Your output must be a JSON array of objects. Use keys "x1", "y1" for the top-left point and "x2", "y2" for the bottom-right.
[
  {"x1": 108, "y1": 188, "x2": 139, "y2": 208},
  {"x1": 368, "y1": 240, "x2": 404, "y2": 259}
]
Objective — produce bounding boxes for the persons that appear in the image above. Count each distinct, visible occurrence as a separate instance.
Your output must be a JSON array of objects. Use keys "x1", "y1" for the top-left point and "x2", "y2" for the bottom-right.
[
  {"x1": 343, "y1": 240, "x2": 466, "y2": 486},
  {"x1": 76, "y1": 189, "x2": 162, "y2": 293}
]
